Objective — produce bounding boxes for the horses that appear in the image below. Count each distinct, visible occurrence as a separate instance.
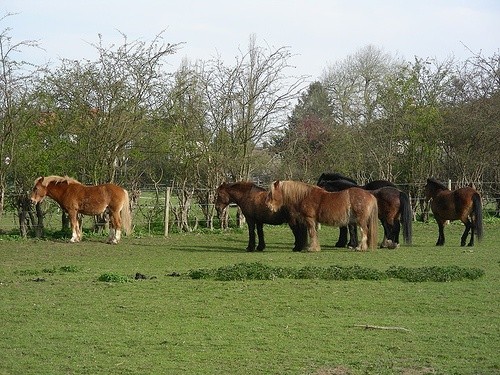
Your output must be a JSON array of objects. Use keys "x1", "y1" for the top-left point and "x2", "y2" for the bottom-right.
[
  {"x1": 30, "y1": 175, "x2": 133, "y2": 245},
  {"x1": 213, "y1": 180, "x2": 304, "y2": 252},
  {"x1": 263, "y1": 181, "x2": 378, "y2": 252},
  {"x1": 424, "y1": 178, "x2": 484, "y2": 248},
  {"x1": 316, "y1": 173, "x2": 414, "y2": 250}
]
